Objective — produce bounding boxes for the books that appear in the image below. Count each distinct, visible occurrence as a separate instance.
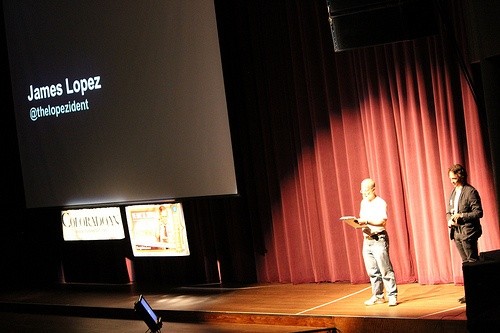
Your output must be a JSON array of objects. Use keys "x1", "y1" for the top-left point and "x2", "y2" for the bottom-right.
[{"x1": 339, "y1": 215, "x2": 369, "y2": 229}]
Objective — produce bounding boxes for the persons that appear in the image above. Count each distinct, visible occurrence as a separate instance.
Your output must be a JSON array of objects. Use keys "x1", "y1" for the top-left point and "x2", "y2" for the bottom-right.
[
  {"x1": 360, "y1": 177, "x2": 398, "y2": 306},
  {"x1": 447, "y1": 163, "x2": 483, "y2": 304}
]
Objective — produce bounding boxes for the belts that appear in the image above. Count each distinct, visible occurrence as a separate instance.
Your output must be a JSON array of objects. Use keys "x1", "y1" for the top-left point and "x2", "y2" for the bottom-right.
[{"x1": 363, "y1": 230, "x2": 386, "y2": 240}]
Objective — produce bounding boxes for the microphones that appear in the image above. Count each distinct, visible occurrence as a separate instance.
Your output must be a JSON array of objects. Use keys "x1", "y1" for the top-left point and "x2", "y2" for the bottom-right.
[
  {"x1": 354, "y1": 220, "x2": 359, "y2": 224},
  {"x1": 450, "y1": 209, "x2": 454, "y2": 223}
]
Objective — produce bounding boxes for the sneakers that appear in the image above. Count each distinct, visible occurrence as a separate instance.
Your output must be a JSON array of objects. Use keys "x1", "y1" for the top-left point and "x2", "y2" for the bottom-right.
[
  {"x1": 387, "y1": 294, "x2": 397, "y2": 306},
  {"x1": 364, "y1": 294, "x2": 385, "y2": 305}
]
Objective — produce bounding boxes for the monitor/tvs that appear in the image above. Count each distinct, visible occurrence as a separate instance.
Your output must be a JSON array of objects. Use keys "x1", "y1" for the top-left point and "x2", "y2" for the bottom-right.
[
  {"x1": 125, "y1": 202, "x2": 191, "y2": 258},
  {"x1": 134, "y1": 294, "x2": 163, "y2": 332},
  {"x1": 60, "y1": 207, "x2": 125, "y2": 240}
]
